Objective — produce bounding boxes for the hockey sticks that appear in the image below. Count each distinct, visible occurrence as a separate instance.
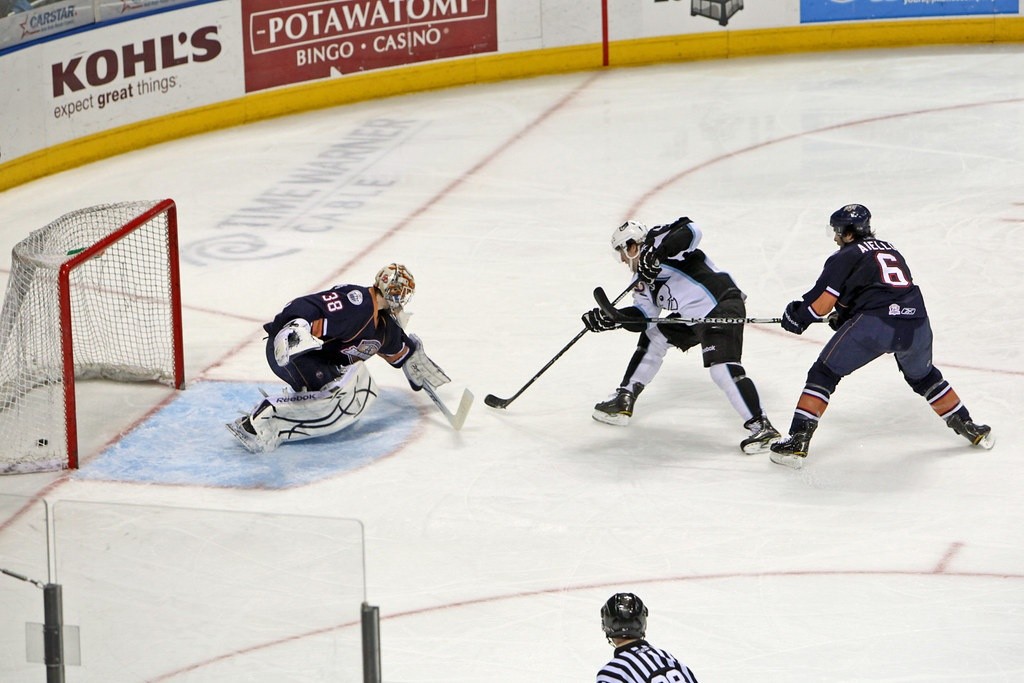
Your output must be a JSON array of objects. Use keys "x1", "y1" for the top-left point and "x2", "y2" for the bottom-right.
[
  {"x1": 483, "y1": 259, "x2": 660, "y2": 409},
  {"x1": 592, "y1": 286, "x2": 831, "y2": 324},
  {"x1": 421, "y1": 379, "x2": 475, "y2": 431}
]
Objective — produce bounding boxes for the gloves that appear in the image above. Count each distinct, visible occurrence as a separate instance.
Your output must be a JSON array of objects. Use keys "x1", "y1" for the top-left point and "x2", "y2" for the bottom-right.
[
  {"x1": 637, "y1": 245, "x2": 663, "y2": 283},
  {"x1": 776, "y1": 300, "x2": 812, "y2": 335},
  {"x1": 581, "y1": 303, "x2": 625, "y2": 333},
  {"x1": 826, "y1": 311, "x2": 838, "y2": 331}
]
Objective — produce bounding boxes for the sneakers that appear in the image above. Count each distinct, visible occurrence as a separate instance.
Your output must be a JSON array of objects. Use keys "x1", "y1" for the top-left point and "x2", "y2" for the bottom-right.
[
  {"x1": 769, "y1": 418, "x2": 818, "y2": 470},
  {"x1": 596, "y1": 381, "x2": 645, "y2": 432},
  {"x1": 740, "y1": 417, "x2": 781, "y2": 453},
  {"x1": 225, "y1": 415, "x2": 273, "y2": 452},
  {"x1": 946, "y1": 413, "x2": 996, "y2": 450}
]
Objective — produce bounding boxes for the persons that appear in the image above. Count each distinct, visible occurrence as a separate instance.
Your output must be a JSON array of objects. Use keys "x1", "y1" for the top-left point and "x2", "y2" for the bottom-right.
[
  {"x1": 226, "y1": 263, "x2": 450, "y2": 452},
  {"x1": 582, "y1": 217, "x2": 782, "y2": 455},
  {"x1": 770, "y1": 204, "x2": 995, "y2": 469},
  {"x1": 597, "y1": 593, "x2": 697, "y2": 683}
]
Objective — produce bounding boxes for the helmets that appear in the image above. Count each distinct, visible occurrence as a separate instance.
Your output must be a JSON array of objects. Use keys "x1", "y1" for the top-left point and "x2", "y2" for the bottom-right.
[
  {"x1": 600, "y1": 592, "x2": 649, "y2": 639},
  {"x1": 610, "y1": 220, "x2": 648, "y2": 251},
  {"x1": 375, "y1": 261, "x2": 416, "y2": 311},
  {"x1": 831, "y1": 204, "x2": 871, "y2": 239}
]
